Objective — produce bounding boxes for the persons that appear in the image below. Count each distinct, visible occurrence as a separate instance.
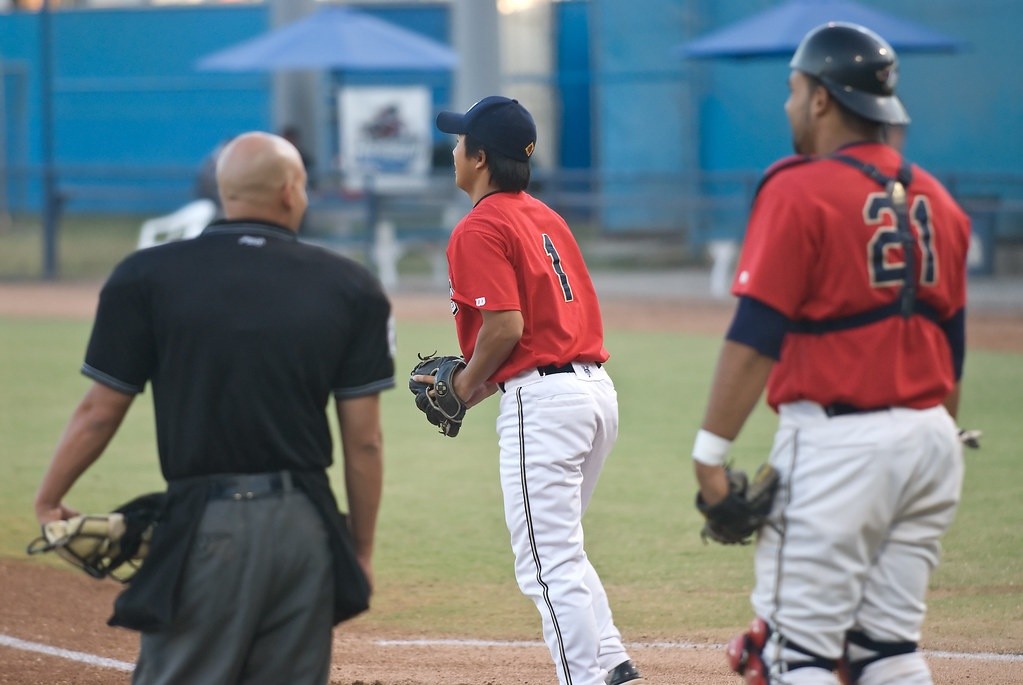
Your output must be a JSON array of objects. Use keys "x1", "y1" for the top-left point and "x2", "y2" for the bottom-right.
[
  {"x1": 408, "y1": 96, "x2": 646, "y2": 685},
  {"x1": 692, "y1": 22, "x2": 981, "y2": 685},
  {"x1": 27, "y1": 132, "x2": 397, "y2": 685}
]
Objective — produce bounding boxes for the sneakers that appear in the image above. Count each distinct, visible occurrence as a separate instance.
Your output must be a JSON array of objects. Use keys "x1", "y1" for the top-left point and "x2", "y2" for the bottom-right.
[{"x1": 605, "y1": 661, "x2": 642, "y2": 685}]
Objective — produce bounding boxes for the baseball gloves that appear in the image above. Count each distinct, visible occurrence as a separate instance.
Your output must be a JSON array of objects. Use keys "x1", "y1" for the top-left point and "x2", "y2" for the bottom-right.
[
  {"x1": 409, "y1": 349, "x2": 468, "y2": 438},
  {"x1": 692, "y1": 454, "x2": 787, "y2": 551},
  {"x1": 27, "y1": 488, "x2": 170, "y2": 586}
]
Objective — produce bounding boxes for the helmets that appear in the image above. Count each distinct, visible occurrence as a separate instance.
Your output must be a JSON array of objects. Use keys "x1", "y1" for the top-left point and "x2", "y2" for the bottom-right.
[{"x1": 790, "y1": 22, "x2": 912, "y2": 126}]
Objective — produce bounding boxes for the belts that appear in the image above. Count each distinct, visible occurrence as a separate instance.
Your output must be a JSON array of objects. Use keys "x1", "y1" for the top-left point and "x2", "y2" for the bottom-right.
[
  {"x1": 207, "y1": 470, "x2": 297, "y2": 498},
  {"x1": 825, "y1": 401, "x2": 890, "y2": 416},
  {"x1": 496, "y1": 360, "x2": 601, "y2": 394}
]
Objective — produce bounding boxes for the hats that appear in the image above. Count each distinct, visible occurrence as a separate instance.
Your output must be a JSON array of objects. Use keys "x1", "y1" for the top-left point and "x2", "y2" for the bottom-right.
[{"x1": 436, "y1": 96, "x2": 536, "y2": 161}]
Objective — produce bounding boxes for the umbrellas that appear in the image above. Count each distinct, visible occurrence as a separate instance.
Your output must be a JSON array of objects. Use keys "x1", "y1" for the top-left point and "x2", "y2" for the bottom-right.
[
  {"x1": 674, "y1": 0, "x2": 964, "y2": 178},
  {"x1": 190, "y1": 5, "x2": 464, "y2": 183}
]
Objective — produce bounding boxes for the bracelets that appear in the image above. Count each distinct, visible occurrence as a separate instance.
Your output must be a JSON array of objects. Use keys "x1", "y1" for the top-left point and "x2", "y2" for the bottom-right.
[{"x1": 691, "y1": 428, "x2": 734, "y2": 467}]
